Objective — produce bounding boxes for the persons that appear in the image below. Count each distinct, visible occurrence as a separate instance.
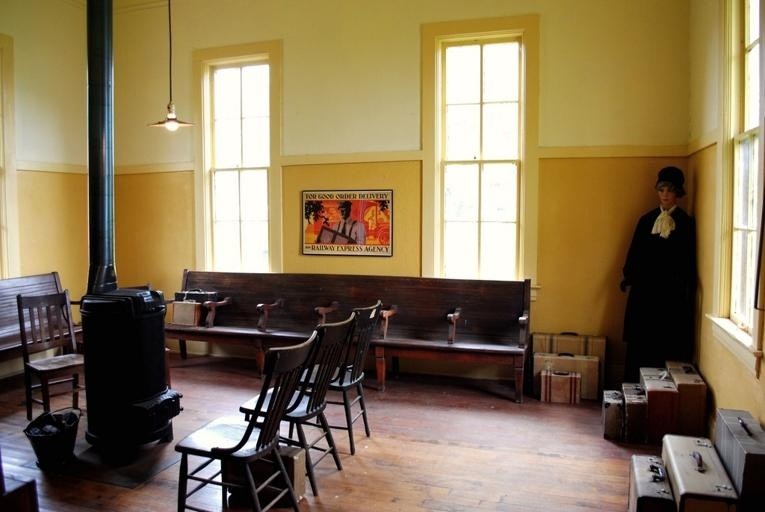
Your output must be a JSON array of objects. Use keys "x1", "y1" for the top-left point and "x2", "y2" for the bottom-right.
[
  {"x1": 333, "y1": 200, "x2": 366, "y2": 246},
  {"x1": 619, "y1": 165, "x2": 700, "y2": 382}
]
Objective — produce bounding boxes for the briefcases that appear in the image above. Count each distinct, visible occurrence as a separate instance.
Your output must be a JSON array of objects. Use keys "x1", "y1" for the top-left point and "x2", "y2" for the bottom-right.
[
  {"x1": 602, "y1": 362, "x2": 710, "y2": 446},
  {"x1": 628, "y1": 408, "x2": 765, "y2": 511},
  {"x1": 530, "y1": 331, "x2": 607, "y2": 404}
]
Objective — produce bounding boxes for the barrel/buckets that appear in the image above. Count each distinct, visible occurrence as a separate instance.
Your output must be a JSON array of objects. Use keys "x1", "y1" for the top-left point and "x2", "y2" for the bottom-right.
[{"x1": 23, "y1": 407, "x2": 82, "y2": 471}]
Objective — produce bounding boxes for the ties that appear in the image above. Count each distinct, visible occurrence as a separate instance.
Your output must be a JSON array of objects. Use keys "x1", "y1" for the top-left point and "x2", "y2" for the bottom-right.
[{"x1": 341, "y1": 222, "x2": 346, "y2": 235}]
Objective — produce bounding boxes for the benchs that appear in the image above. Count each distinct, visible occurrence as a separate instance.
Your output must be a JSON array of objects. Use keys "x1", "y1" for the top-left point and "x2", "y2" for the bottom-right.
[
  {"x1": 0, "y1": 272, "x2": 85, "y2": 360},
  {"x1": 159, "y1": 270, "x2": 531, "y2": 402}
]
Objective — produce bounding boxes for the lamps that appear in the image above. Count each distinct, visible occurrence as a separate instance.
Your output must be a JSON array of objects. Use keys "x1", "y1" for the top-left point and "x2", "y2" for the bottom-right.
[{"x1": 148, "y1": 0, "x2": 196, "y2": 131}]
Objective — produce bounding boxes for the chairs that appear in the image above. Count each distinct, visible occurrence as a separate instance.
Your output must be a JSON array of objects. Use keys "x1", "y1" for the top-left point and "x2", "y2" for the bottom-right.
[
  {"x1": 2, "y1": 451, "x2": 41, "y2": 511},
  {"x1": 256, "y1": 312, "x2": 356, "y2": 496},
  {"x1": 300, "y1": 300, "x2": 383, "y2": 436},
  {"x1": 175, "y1": 330, "x2": 317, "y2": 512},
  {"x1": 17, "y1": 289, "x2": 85, "y2": 421}
]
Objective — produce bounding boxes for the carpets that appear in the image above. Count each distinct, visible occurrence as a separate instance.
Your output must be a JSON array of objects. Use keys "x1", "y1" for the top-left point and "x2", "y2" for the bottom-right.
[{"x1": 20, "y1": 405, "x2": 228, "y2": 488}]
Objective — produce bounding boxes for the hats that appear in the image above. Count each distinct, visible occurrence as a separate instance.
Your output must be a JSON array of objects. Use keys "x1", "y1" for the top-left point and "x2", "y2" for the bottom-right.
[
  {"x1": 654, "y1": 166, "x2": 686, "y2": 197},
  {"x1": 337, "y1": 199, "x2": 353, "y2": 211}
]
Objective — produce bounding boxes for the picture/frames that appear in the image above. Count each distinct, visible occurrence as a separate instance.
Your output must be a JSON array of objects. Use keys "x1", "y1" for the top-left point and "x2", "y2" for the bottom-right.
[{"x1": 300, "y1": 190, "x2": 393, "y2": 256}]
